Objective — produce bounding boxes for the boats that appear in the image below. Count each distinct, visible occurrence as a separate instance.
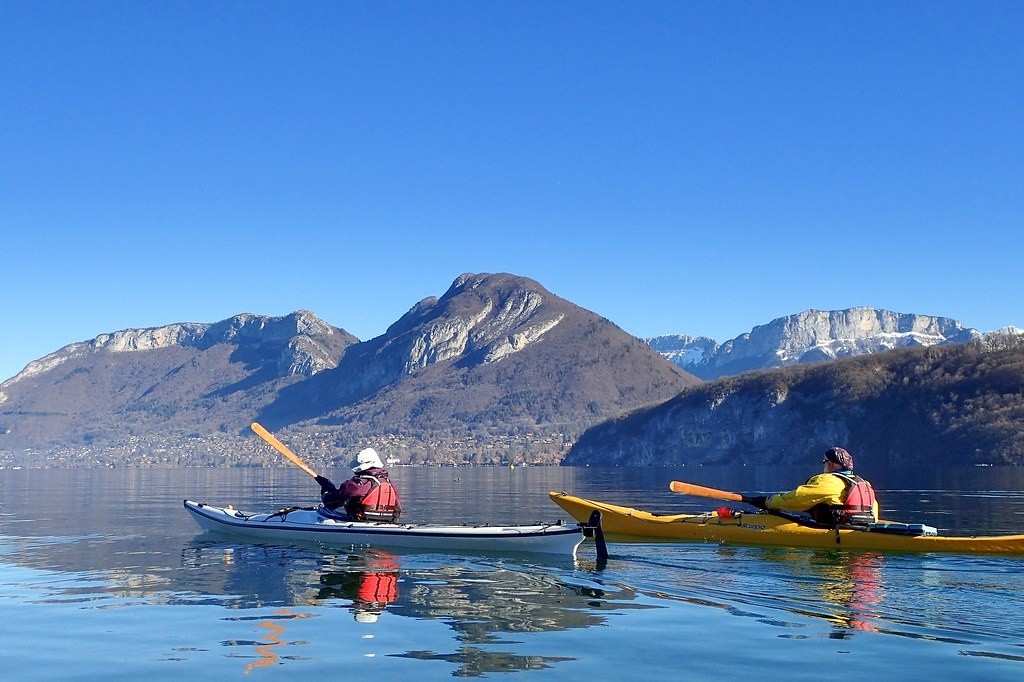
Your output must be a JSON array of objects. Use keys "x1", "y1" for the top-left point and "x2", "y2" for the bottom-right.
[
  {"x1": 549, "y1": 488, "x2": 1024, "y2": 554},
  {"x1": 182, "y1": 497, "x2": 611, "y2": 559}
]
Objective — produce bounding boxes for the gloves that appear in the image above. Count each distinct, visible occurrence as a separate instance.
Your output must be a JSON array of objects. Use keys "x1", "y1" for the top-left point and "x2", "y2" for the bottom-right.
[
  {"x1": 822, "y1": 459, "x2": 829, "y2": 464},
  {"x1": 320, "y1": 479, "x2": 335, "y2": 494},
  {"x1": 750, "y1": 496, "x2": 768, "y2": 510}
]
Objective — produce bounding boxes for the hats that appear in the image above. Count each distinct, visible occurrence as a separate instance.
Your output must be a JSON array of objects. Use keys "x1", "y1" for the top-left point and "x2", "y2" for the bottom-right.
[
  {"x1": 350, "y1": 448, "x2": 384, "y2": 472},
  {"x1": 825, "y1": 446, "x2": 853, "y2": 471},
  {"x1": 350, "y1": 609, "x2": 382, "y2": 623}
]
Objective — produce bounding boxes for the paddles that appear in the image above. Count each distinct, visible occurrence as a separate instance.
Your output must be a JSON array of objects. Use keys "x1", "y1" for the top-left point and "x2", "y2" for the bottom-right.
[
  {"x1": 250, "y1": 423, "x2": 334, "y2": 487},
  {"x1": 668, "y1": 479, "x2": 784, "y2": 511}
]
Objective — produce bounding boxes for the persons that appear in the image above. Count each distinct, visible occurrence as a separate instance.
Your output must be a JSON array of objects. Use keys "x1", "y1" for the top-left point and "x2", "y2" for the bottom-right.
[
  {"x1": 315, "y1": 448, "x2": 402, "y2": 524},
  {"x1": 316, "y1": 552, "x2": 399, "y2": 623},
  {"x1": 751, "y1": 447, "x2": 879, "y2": 528}
]
